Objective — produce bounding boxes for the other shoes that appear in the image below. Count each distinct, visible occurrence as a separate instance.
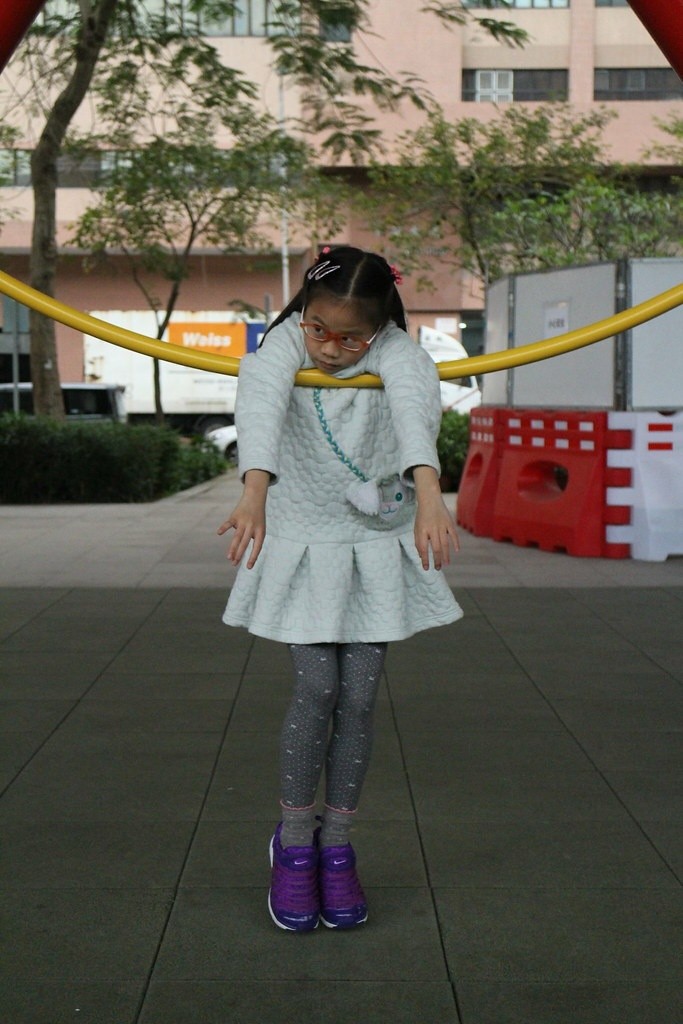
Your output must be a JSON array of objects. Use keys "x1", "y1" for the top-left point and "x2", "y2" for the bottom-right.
[
  {"x1": 268, "y1": 820, "x2": 319, "y2": 933},
  {"x1": 314, "y1": 826, "x2": 369, "y2": 930}
]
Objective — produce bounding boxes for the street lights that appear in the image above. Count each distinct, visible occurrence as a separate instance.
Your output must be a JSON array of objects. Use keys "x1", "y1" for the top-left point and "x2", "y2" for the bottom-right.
[{"x1": 268, "y1": 59, "x2": 292, "y2": 308}]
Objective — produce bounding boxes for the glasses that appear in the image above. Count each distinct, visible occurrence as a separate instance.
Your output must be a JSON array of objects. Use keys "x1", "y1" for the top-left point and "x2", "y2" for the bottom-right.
[{"x1": 300, "y1": 304, "x2": 382, "y2": 352}]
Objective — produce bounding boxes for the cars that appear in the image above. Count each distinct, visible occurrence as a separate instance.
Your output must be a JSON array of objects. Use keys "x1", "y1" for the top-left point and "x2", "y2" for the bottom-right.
[{"x1": 204, "y1": 421, "x2": 240, "y2": 468}]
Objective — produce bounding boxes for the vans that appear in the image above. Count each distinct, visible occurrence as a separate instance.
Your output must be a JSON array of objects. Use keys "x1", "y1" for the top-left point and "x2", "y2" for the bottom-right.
[{"x1": 0, "y1": 382, "x2": 128, "y2": 430}]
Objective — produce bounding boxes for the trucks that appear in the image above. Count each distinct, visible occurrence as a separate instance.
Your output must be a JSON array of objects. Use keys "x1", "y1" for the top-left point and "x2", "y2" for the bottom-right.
[{"x1": 82, "y1": 309, "x2": 482, "y2": 438}]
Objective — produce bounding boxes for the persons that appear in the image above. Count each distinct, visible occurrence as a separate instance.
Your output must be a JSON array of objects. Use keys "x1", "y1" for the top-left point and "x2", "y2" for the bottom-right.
[{"x1": 215, "y1": 244, "x2": 464, "y2": 932}]
